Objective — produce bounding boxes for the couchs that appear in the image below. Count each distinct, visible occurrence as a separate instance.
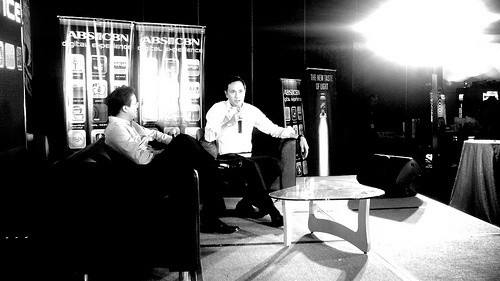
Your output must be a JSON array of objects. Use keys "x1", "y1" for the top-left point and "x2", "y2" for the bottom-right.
[
  {"x1": 62, "y1": 137, "x2": 202, "y2": 281},
  {"x1": 196, "y1": 128, "x2": 298, "y2": 246}
]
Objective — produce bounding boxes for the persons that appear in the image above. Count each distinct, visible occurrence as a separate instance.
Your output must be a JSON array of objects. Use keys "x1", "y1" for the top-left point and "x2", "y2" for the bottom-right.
[
  {"x1": 361, "y1": 93, "x2": 382, "y2": 127},
  {"x1": 104, "y1": 85, "x2": 241, "y2": 233},
  {"x1": 204, "y1": 77, "x2": 309, "y2": 227}
]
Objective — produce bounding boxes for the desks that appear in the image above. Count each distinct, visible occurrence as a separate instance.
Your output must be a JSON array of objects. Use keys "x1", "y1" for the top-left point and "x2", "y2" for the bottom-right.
[{"x1": 450, "y1": 140, "x2": 500, "y2": 227}]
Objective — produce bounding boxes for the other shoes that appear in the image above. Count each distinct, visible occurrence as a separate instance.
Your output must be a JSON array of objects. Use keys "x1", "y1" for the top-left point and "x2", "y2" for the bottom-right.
[
  {"x1": 200, "y1": 219, "x2": 240, "y2": 234},
  {"x1": 236, "y1": 200, "x2": 255, "y2": 211},
  {"x1": 270, "y1": 210, "x2": 283, "y2": 226}
]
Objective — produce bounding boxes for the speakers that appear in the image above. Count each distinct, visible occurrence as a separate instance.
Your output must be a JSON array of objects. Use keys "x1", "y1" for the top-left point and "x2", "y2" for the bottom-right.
[{"x1": 358, "y1": 153, "x2": 420, "y2": 198}]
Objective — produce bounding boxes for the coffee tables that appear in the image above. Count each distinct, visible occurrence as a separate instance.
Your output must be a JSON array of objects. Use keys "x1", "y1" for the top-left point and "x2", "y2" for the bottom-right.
[{"x1": 268, "y1": 174, "x2": 385, "y2": 254}]
